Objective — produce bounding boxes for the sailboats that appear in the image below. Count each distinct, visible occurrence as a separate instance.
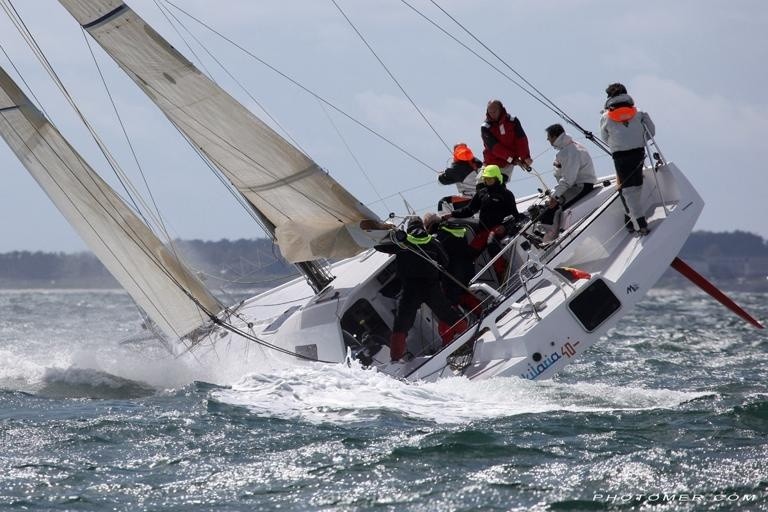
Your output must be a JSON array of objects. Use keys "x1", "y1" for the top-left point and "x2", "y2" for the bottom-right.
[{"x1": 0, "y1": 0, "x2": 712, "y2": 386}]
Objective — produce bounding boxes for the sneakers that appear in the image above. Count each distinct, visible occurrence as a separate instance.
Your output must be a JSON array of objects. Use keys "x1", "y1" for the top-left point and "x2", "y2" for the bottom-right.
[{"x1": 637, "y1": 227, "x2": 650, "y2": 236}]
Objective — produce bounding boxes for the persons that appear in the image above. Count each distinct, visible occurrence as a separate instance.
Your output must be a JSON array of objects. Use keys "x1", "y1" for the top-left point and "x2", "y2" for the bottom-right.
[
  {"x1": 539, "y1": 123, "x2": 598, "y2": 242},
  {"x1": 374, "y1": 214, "x2": 474, "y2": 361},
  {"x1": 438, "y1": 142, "x2": 483, "y2": 213},
  {"x1": 599, "y1": 82, "x2": 656, "y2": 237},
  {"x1": 445, "y1": 163, "x2": 522, "y2": 285},
  {"x1": 476, "y1": 99, "x2": 534, "y2": 192},
  {"x1": 423, "y1": 210, "x2": 483, "y2": 346}
]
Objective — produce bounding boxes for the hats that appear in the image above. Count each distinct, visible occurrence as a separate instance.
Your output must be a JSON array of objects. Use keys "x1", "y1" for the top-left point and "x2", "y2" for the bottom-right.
[{"x1": 482, "y1": 165, "x2": 503, "y2": 185}]
